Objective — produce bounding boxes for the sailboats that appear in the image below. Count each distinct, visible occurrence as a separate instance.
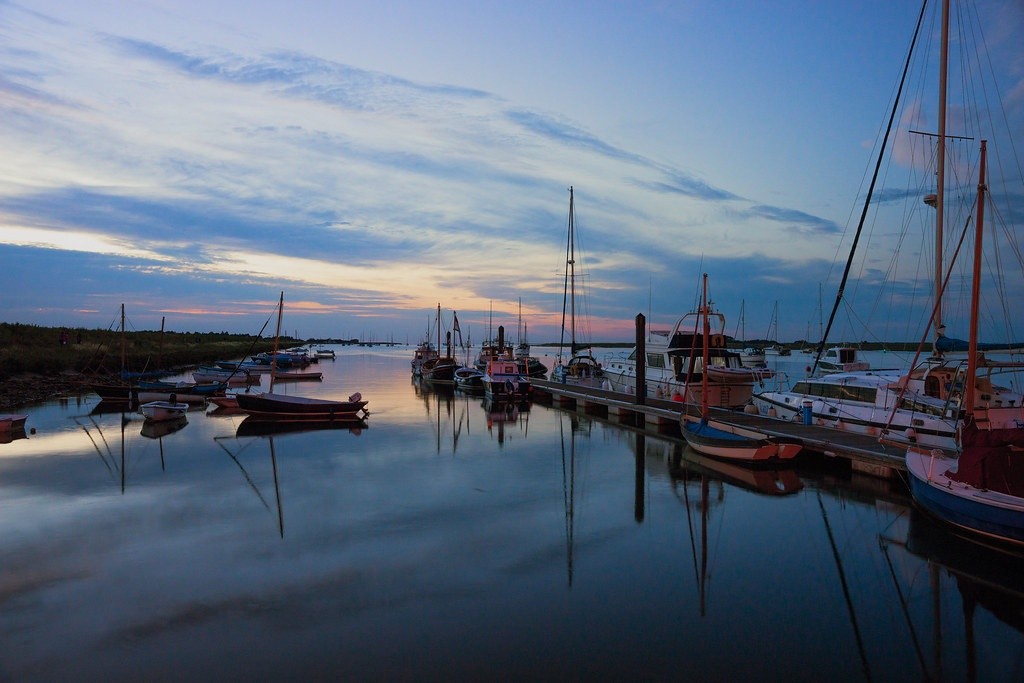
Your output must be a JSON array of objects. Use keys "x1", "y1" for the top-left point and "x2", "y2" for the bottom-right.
[
  {"x1": 410, "y1": 297, "x2": 549, "y2": 413},
  {"x1": 756, "y1": 0, "x2": 1023, "y2": 457},
  {"x1": 550, "y1": 185, "x2": 605, "y2": 388},
  {"x1": 89, "y1": 300, "x2": 230, "y2": 403},
  {"x1": 879, "y1": 501, "x2": 1024, "y2": 683}
]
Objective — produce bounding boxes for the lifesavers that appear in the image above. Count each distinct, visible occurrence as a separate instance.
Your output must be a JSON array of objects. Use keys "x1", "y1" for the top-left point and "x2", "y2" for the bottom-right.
[{"x1": 712, "y1": 333, "x2": 723, "y2": 347}]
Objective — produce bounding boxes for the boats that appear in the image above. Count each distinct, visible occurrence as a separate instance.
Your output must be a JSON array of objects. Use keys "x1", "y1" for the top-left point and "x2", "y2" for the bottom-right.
[
  {"x1": 872, "y1": 137, "x2": 1024, "y2": 548},
  {"x1": 777, "y1": 345, "x2": 792, "y2": 356},
  {"x1": 815, "y1": 346, "x2": 871, "y2": 374},
  {"x1": 191, "y1": 370, "x2": 261, "y2": 385},
  {"x1": 205, "y1": 291, "x2": 284, "y2": 407},
  {"x1": 215, "y1": 347, "x2": 335, "y2": 371},
  {"x1": 676, "y1": 272, "x2": 804, "y2": 464},
  {"x1": 236, "y1": 414, "x2": 371, "y2": 438},
  {"x1": 274, "y1": 372, "x2": 324, "y2": 379},
  {"x1": 139, "y1": 393, "x2": 190, "y2": 421},
  {"x1": 728, "y1": 297, "x2": 776, "y2": 379},
  {"x1": 603, "y1": 274, "x2": 777, "y2": 409},
  {"x1": 198, "y1": 364, "x2": 249, "y2": 374},
  {"x1": 140, "y1": 416, "x2": 189, "y2": 438},
  {"x1": 234, "y1": 390, "x2": 369, "y2": 416},
  {"x1": 0, "y1": 413, "x2": 30, "y2": 432},
  {"x1": 678, "y1": 444, "x2": 805, "y2": 622}
]
